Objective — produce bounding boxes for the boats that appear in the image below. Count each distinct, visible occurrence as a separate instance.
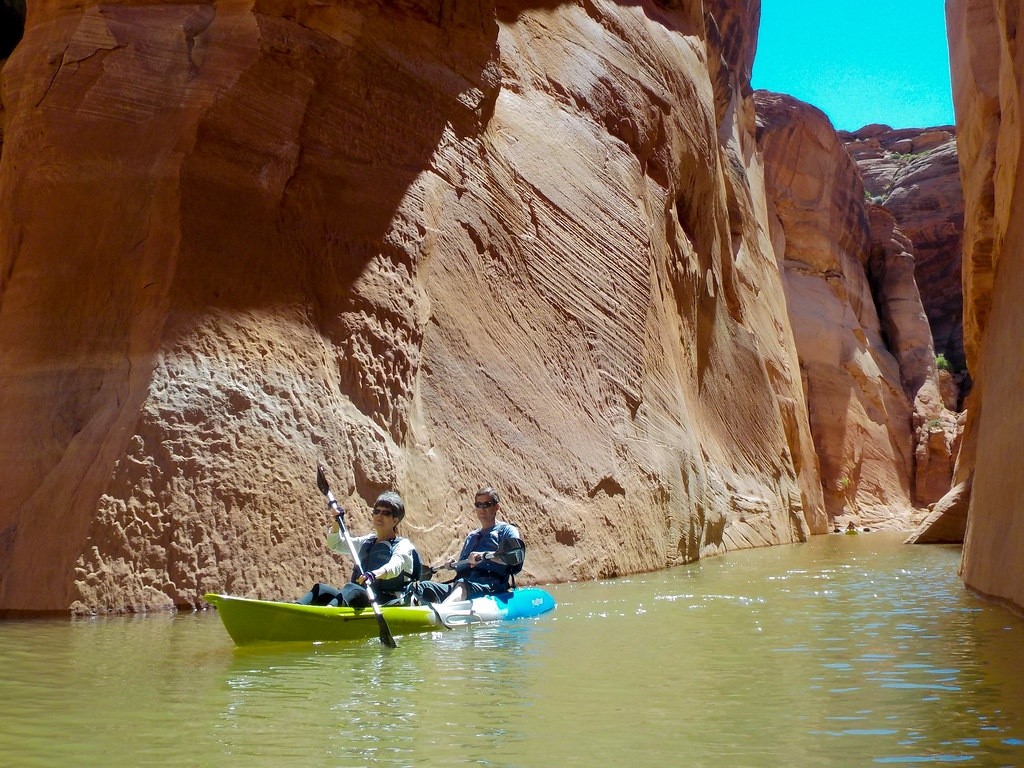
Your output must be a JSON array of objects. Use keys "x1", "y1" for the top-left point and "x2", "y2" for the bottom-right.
[{"x1": 202, "y1": 584, "x2": 559, "y2": 646}]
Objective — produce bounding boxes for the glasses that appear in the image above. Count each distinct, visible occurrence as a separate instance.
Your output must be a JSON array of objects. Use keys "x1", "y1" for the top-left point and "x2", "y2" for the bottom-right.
[
  {"x1": 372, "y1": 509, "x2": 393, "y2": 516},
  {"x1": 474, "y1": 502, "x2": 494, "y2": 508}
]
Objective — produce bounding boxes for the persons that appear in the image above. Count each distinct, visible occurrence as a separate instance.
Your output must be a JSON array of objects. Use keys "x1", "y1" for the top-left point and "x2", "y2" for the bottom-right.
[
  {"x1": 402, "y1": 488, "x2": 527, "y2": 605},
  {"x1": 294, "y1": 490, "x2": 415, "y2": 607}
]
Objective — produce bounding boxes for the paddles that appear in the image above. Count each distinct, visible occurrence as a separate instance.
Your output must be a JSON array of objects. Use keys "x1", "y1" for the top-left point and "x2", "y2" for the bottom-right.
[
  {"x1": 420, "y1": 537, "x2": 526, "y2": 583},
  {"x1": 314, "y1": 460, "x2": 398, "y2": 650}
]
